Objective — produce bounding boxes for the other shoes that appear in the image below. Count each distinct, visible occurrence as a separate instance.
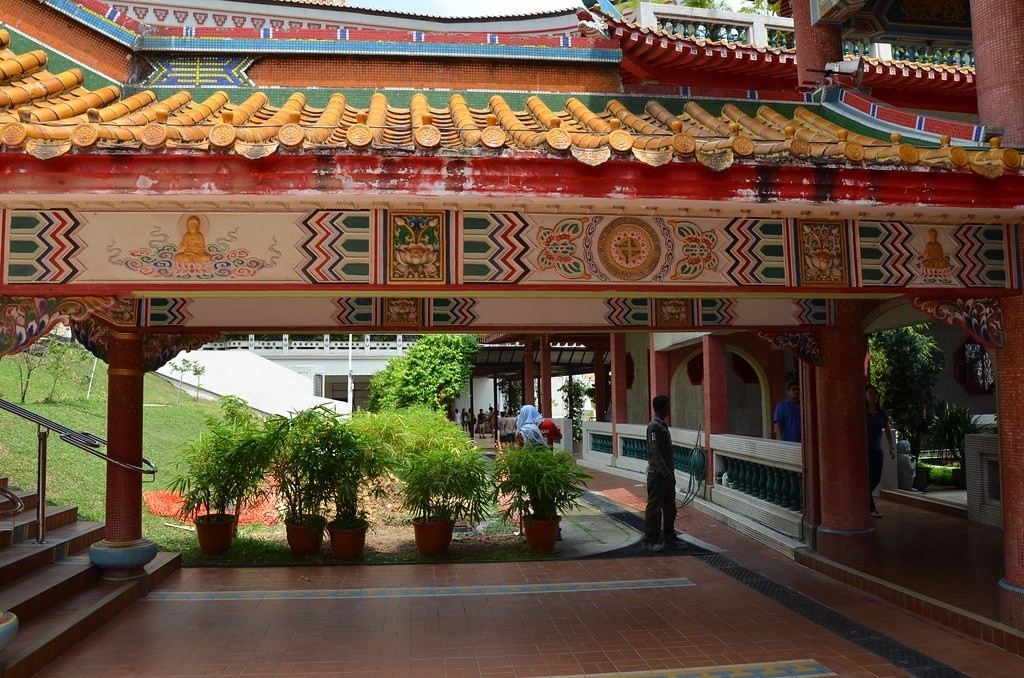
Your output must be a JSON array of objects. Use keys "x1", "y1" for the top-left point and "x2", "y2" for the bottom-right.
[
  {"x1": 663, "y1": 541, "x2": 689, "y2": 551},
  {"x1": 642, "y1": 540, "x2": 662, "y2": 553},
  {"x1": 870, "y1": 509, "x2": 882, "y2": 518},
  {"x1": 480, "y1": 436, "x2": 482, "y2": 439},
  {"x1": 483, "y1": 436, "x2": 486, "y2": 438}
]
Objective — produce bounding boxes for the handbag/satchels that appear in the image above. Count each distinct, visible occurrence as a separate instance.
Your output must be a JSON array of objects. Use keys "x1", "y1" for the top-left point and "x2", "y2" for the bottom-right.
[
  {"x1": 475, "y1": 425, "x2": 480, "y2": 432},
  {"x1": 473, "y1": 418, "x2": 476, "y2": 424}
]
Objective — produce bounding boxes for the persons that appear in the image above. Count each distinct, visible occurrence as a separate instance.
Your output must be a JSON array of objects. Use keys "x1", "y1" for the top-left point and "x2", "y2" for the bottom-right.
[
  {"x1": 641, "y1": 394, "x2": 689, "y2": 552},
  {"x1": 518, "y1": 405, "x2": 562, "y2": 452},
  {"x1": 455, "y1": 407, "x2": 520, "y2": 446},
  {"x1": 865, "y1": 384, "x2": 896, "y2": 518},
  {"x1": 897, "y1": 440, "x2": 917, "y2": 492},
  {"x1": 775, "y1": 380, "x2": 801, "y2": 444}
]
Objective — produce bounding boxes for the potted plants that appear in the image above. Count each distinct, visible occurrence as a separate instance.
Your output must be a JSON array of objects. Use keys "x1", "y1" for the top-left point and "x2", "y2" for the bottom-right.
[
  {"x1": 160, "y1": 395, "x2": 593, "y2": 561},
  {"x1": 872, "y1": 327, "x2": 949, "y2": 493},
  {"x1": 925, "y1": 402, "x2": 991, "y2": 490}
]
